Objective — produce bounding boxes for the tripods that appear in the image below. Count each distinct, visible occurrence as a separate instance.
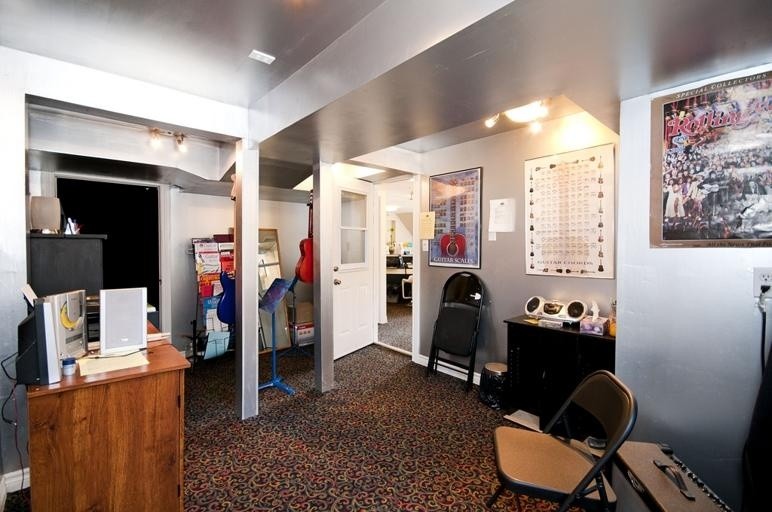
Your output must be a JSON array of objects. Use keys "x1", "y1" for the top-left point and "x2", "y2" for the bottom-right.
[
  {"x1": 280, "y1": 295, "x2": 313, "y2": 358},
  {"x1": 258, "y1": 311, "x2": 296, "y2": 395}
]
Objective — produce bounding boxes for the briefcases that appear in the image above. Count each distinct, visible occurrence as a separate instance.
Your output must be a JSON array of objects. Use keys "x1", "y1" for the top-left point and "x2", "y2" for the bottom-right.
[{"x1": 611, "y1": 441, "x2": 732, "y2": 512}]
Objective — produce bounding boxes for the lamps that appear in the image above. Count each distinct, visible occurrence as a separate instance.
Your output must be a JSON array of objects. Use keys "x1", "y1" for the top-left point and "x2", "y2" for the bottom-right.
[
  {"x1": 147, "y1": 126, "x2": 187, "y2": 154},
  {"x1": 483, "y1": 97, "x2": 552, "y2": 136}
]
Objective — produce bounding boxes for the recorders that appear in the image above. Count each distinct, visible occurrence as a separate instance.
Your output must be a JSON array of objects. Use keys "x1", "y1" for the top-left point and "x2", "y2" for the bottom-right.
[{"x1": 524, "y1": 295, "x2": 588, "y2": 324}]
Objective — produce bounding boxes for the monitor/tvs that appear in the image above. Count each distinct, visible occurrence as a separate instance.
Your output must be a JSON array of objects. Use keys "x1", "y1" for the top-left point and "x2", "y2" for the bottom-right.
[
  {"x1": 386, "y1": 255, "x2": 401, "y2": 268},
  {"x1": 402, "y1": 256, "x2": 413, "y2": 268}
]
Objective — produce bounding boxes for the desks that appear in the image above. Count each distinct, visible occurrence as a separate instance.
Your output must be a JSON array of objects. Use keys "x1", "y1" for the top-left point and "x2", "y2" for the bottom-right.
[{"x1": 387, "y1": 266, "x2": 414, "y2": 302}]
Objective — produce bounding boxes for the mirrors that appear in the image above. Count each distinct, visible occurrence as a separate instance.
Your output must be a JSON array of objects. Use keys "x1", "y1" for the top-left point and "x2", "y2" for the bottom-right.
[{"x1": 228, "y1": 227, "x2": 293, "y2": 356}]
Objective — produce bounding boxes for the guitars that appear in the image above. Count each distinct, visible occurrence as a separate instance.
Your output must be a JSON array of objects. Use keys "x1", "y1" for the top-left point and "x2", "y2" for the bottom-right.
[
  {"x1": 217, "y1": 175, "x2": 235, "y2": 324},
  {"x1": 296, "y1": 190, "x2": 313, "y2": 284}
]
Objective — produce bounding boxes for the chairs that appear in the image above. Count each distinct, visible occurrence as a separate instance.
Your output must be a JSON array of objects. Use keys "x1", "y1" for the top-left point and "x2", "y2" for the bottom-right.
[
  {"x1": 486, "y1": 364, "x2": 641, "y2": 511},
  {"x1": 426, "y1": 269, "x2": 488, "y2": 391},
  {"x1": 401, "y1": 275, "x2": 413, "y2": 307}
]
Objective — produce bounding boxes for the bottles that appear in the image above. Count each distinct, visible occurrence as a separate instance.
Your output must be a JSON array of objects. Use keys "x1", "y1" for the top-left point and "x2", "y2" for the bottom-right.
[
  {"x1": 607, "y1": 303, "x2": 615, "y2": 337},
  {"x1": 61, "y1": 357, "x2": 77, "y2": 375}
]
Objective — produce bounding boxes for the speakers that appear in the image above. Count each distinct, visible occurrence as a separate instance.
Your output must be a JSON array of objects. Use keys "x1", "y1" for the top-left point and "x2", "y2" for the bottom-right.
[
  {"x1": 27, "y1": 196, "x2": 61, "y2": 234},
  {"x1": 99, "y1": 287, "x2": 148, "y2": 355}
]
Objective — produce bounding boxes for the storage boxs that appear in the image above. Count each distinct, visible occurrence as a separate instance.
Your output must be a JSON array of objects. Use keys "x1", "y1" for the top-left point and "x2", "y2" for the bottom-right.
[{"x1": 288, "y1": 322, "x2": 315, "y2": 347}]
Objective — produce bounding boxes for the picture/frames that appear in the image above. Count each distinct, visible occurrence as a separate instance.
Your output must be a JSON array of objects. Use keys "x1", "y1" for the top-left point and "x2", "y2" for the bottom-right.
[{"x1": 428, "y1": 166, "x2": 482, "y2": 268}]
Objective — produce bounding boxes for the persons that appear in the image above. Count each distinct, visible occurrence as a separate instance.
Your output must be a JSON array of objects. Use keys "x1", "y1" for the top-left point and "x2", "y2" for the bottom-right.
[{"x1": 662, "y1": 80, "x2": 771, "y2": 239}]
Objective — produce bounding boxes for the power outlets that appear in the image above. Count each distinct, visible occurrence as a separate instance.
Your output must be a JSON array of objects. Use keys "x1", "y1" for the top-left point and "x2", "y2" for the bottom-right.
[{"x1": 752, "y1": 264, "x2": 772, "y2": 303}]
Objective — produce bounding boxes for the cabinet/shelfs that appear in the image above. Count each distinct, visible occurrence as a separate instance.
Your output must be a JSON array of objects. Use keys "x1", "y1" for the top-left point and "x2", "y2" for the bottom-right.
[
  {"x1": 27, "y1": 228, "x2": 108, "y2": 300},
  {"x1": 185, "y1": 233, "x2": 240, "y2": 367},
  {"x1": 501, "y1": 311, "x2": 615, "y2": 417},
  {"x1": 25, "y1": 317, "x2": 191, "y2": 512}
]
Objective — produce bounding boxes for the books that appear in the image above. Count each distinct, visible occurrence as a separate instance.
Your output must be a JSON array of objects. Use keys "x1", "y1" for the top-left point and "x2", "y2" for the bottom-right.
[{"x1": 193, "y1": 241, "x2": 236, "y2": 360}]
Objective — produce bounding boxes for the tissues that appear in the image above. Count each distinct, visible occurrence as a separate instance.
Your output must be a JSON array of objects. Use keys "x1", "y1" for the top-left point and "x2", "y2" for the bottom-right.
[{"x1": 579, "y1": 301, "x2": 609, "y2": 336}]
